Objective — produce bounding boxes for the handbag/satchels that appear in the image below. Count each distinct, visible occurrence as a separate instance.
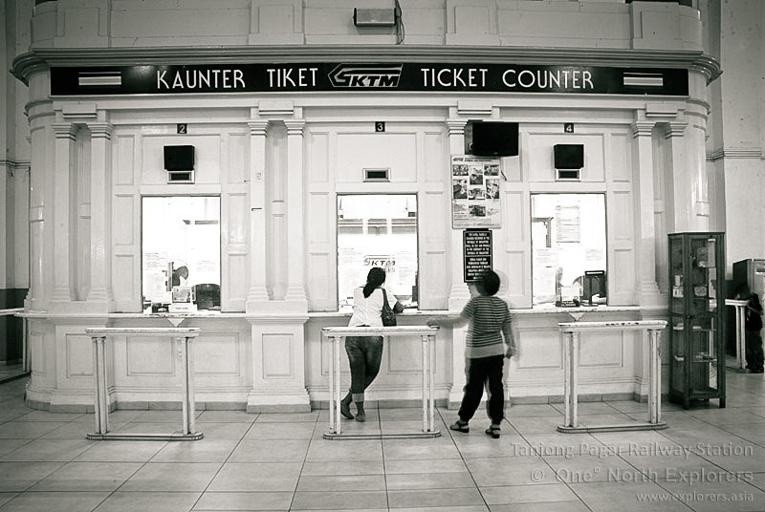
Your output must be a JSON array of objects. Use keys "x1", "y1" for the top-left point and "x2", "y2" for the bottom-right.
[{"x1": 381, "y1": 288, "x2": 396, "y2": 326}]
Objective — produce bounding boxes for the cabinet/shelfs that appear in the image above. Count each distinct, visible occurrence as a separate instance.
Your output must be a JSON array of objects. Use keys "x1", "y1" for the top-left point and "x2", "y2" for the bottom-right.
[{"x1": 667, "y1": 232, "x2": 725, "y2": 410}]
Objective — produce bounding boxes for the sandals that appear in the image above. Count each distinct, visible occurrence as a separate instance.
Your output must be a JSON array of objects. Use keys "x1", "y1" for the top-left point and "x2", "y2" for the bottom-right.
[
  {"x1": 450, "y1": 421, "x2": 469, "y2": 433},
  {"x1": 484, "y1": 427, "x2": 501, "y2": 438}
]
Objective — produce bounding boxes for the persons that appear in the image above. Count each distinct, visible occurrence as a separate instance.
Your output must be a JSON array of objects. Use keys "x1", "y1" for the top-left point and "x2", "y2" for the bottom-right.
[
  {"x1": 332, "y1": 266, "x2": 405, "y2": 424},
  {"x1": 426, "y1": 266, "x2": 515, "y2": 440}
]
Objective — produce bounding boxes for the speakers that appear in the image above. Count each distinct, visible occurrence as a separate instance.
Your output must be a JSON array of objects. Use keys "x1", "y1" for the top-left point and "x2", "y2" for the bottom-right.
[
  {"x1": 354, "y1": 8, "x2": 395, "y2": 26},
  {"x1": 554, "y1": 144, "x2": 585, "y2": 169},
  {"x1": 464, "y1": 121, "x2": 519, "y2": 157},
  {"x1": 163, "y1": 145, "x2": 195, "y2": 171}
]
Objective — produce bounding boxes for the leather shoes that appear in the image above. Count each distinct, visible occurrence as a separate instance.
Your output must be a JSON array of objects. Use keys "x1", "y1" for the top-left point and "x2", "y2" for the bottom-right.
[
  {"x1": 341, "y1": 402, "x2": 354, "y2": 419},
  {"x1": 356, "y1": 412, "x2": 366, "y2": 421}
]
